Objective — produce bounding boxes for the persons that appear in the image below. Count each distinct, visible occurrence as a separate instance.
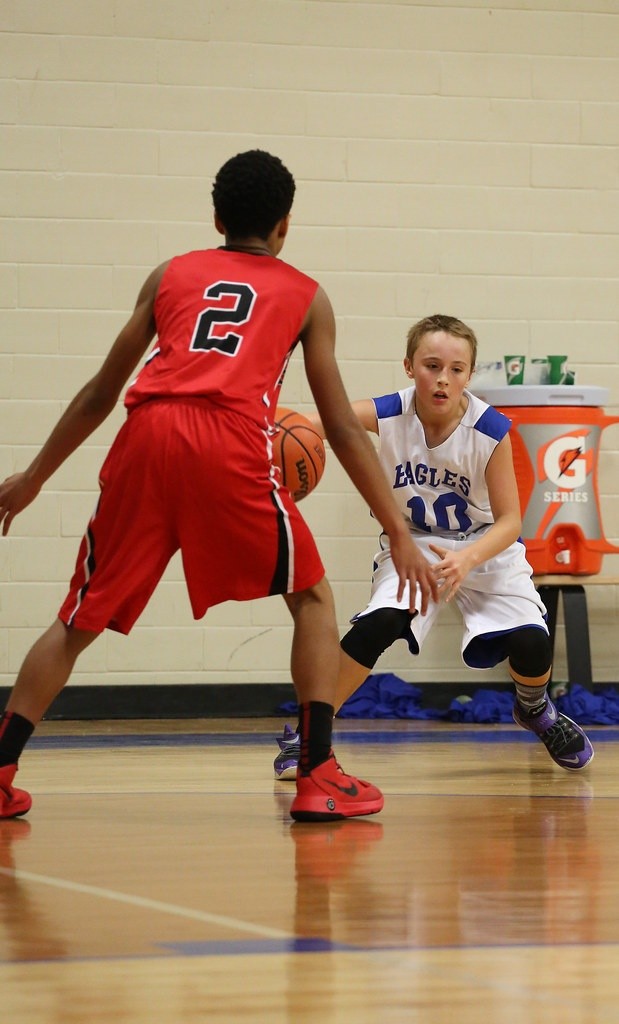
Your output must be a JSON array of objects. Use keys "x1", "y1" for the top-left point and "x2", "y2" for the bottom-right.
[
  {"x1": 274, "y1": 314, "x2": 594, "y2": 779},
  {"x1": 0, "y1": 149, "x2": 439, "y2": 819}
]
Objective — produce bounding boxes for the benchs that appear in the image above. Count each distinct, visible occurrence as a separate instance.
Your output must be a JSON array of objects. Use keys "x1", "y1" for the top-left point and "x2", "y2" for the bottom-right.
[{"x1": 531, "y1": 574, "x2": 618, "y2": 698}]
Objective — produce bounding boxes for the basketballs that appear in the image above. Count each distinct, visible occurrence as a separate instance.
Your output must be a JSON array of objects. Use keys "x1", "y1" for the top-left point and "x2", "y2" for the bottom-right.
[{"x1": 271, "y1": 406, "x2": 327, "y2": 503}]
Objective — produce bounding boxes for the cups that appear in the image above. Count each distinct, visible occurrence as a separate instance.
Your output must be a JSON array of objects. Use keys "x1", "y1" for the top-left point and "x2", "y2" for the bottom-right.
[
  {"x1": 505, "y1": 355, "x2": 526, "y2": 384},
  {"x1": 548, "y1": 355, "x2": 567, "y2": 385}
]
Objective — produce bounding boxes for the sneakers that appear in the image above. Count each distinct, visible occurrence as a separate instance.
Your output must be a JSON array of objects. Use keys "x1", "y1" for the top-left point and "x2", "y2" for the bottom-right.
[
  {"x1": 0, "y1": 762, "x2": 33, "y2": 819},
  {"x1": 513, "y1": 692, "x2": 595, "y2": 772},
  {"x1": 289, "y1": 723, "x2": 385, "y2": 822},
  {"x1": 273, "y1": 724, "x2": 300, "y2": 780}
]
